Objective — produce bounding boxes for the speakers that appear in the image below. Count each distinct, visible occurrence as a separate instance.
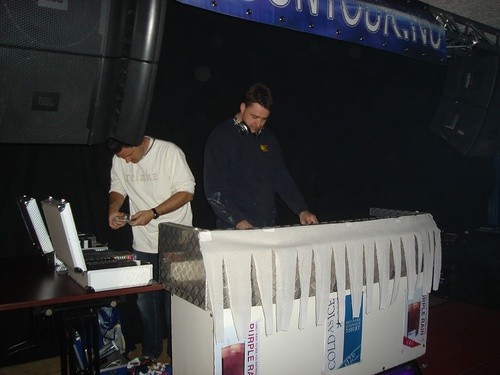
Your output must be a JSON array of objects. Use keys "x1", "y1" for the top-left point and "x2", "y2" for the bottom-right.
[
  {"x1": 428, "y1": 53, "x2": 500, "y2": 158},
  {"x1": 1, "y1": 56, "x2": 159, "y2": 145}
]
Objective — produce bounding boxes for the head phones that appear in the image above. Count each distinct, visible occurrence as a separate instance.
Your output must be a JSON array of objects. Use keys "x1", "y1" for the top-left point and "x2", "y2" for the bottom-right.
[{"x1": 232, "y1": 114, "x2": 265, "y2": 138}]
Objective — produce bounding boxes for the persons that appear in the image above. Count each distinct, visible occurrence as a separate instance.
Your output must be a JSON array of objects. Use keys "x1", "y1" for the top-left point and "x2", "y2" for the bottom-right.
[
  {"x1": 108, "y1": 123, "x2": 196, "y2": 362},
  {"x1": 203, "y1": 85, "x2": 319, "y2": 230}
]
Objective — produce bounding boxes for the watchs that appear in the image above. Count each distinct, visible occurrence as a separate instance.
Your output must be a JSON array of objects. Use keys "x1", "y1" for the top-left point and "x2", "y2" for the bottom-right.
[{"x1": 151, "y1": 208, "x2": 159, "y2": 219}]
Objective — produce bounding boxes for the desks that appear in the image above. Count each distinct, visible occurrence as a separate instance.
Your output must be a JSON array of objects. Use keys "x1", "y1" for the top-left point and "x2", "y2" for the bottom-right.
[{"x1": 1, "y1": 258, "x2": 165, "y2": 375}]
